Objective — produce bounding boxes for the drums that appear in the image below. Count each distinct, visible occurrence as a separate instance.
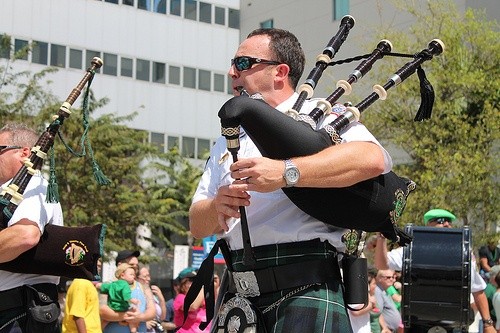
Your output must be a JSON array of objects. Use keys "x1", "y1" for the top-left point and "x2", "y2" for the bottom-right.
[{"x1": 401, "y1": 226, "x2": 474, "y2": 330}]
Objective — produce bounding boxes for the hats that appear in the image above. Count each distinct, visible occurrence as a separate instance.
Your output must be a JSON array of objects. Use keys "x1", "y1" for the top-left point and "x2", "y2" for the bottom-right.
[
  {"x1": 423, "y1": 209, "x2": 457, "y2": 224},
  {"x1": 115, "y1": 249, "x2": 140, "y2": 265},
  {"x1": 115, "y1": 263, "x2": 136, "y2": 278},
  {"x1": 178, "y1": 268, "x2": 198, "y2": 280}
]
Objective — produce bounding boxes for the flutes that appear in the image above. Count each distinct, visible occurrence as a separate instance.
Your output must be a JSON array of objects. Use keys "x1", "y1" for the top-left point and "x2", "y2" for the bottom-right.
[
  {"x1": 210, "y1": 15, "x2": 445, "y2": 264},
  {"x1": 0, "y1": 57, "x2": 104, "y2": 231}
]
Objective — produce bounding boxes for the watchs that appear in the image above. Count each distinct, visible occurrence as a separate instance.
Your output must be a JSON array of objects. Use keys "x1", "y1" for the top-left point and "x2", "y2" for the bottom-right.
[{"x1": 283, "y1": 160, "x2": 300, "y2": 187}]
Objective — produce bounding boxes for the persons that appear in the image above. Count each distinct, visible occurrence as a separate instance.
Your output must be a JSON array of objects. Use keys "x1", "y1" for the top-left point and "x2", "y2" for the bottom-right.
[
  {"x1": 62, "y1": 250, "x2": 221, "y2": 333},
  {"x1": 189, "y1": 28, "x2": 394, "y2": 333},
  {"x1": 479, "y1": 241, "x2": 500, "y2": 333},
  {"x1": 375, "y1": 209, "x2": 498, "y2": 333},
  {"x1": 0, "y1": 122, "x2": 61, "y2": 333},
  {"x1": 347, "y1": 269, "x2": 402, "y2": 333}
]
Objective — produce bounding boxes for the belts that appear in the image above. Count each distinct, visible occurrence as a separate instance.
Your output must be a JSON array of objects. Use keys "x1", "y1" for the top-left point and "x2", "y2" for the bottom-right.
[
  {"x1": 0, "y1": 284, "x2": 58, "y2": 312},
  {"x1": 228, "y1": 260, "x2": 341, "y2": 298}
]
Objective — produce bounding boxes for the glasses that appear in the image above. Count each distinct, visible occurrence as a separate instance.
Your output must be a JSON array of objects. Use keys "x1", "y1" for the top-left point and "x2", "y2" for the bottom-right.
[
  {"x1": 231, "y1": 56, "x2": 281, "y2": 71},
  {"x1": 138, "y1": 273, "x2": 152, "y2": 280},
  {"x1": 429, "y1": 218, "x2": 452, "y2": 224},
  {"x1": 385, "y1": 276, "x2": 394, "y2": 279}
]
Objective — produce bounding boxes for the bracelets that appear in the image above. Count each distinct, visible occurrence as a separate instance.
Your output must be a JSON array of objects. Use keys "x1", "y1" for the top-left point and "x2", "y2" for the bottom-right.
[{"x1": 483, "y1": 319, "x2": 494, "y2": 328}]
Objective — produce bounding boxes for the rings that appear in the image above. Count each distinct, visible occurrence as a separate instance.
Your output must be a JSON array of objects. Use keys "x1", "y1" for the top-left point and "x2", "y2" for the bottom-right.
[{"x1": 246, "y1": 178, "x2": 249, "y2": 185}]
